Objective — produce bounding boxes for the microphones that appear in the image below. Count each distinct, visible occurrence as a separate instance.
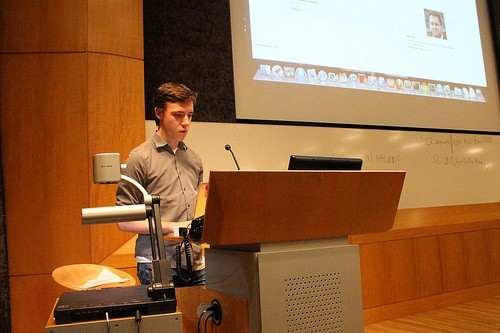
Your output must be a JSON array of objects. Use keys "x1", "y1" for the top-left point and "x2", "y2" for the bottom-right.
[{"x1": 225, "y1": 145, "x2": 240, "y2": 170}]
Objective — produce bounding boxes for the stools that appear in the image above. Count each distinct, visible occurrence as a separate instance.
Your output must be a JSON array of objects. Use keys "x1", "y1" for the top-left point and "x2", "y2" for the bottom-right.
[{"x1": 51, "y1": 264, "x2": 137, "y2": 294}]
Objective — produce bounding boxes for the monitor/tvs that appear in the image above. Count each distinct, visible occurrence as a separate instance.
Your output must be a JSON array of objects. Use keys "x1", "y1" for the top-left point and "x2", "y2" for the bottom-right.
[{"x1": 288, "y1": 155, "x2": 362, "y2": 171}]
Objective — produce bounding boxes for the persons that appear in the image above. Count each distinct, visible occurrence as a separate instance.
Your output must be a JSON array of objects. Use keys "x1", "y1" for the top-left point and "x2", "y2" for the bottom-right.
[
  {"x1": 427, "y1": 13, "x2": 447, "y2": 39},
  {"x1": 115, "y1": 82, "x2": 207, "y2": 288}
]
